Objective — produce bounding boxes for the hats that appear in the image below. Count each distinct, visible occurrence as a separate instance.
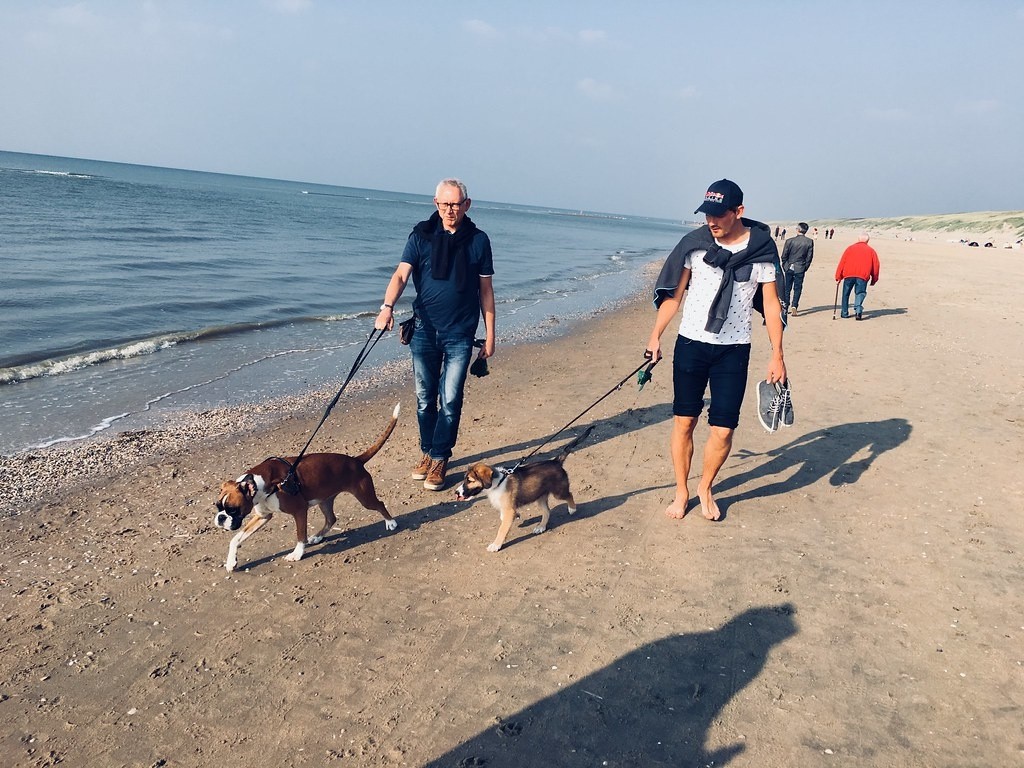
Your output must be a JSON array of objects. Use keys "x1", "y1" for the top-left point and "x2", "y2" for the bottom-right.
[{"x1": 694, "y1": 179, "x2": 744, "y2": 216}]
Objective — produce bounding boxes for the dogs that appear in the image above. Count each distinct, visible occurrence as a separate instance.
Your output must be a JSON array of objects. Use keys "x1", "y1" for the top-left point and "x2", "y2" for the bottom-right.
[
  {"x1": 454, "y1": 426, "x2": 595, "y2": 552},
  {"x1": 214, "y1": 402, "x2": 401, "y2": 573}
]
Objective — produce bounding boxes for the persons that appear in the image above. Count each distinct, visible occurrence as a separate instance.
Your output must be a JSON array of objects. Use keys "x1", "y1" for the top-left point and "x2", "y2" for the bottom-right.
[
  {"x1": 645, "y1": 178, "x2": 788, "y2": 523},
  {"x1": 774, "y1": 225, "x2": 786, "y2": 241},
  {"x1": 812, "y1": 227, "x2": 835, "y2": 240},
  {"x1": 780, "y1": 222, "x2": 814, "y2": 316},
  {"x1": 374, "y1": 178, "x2": 496, "y2": 490},
  {"x1": 835, "y1": 233, "x2": 880, "y2": 321}
]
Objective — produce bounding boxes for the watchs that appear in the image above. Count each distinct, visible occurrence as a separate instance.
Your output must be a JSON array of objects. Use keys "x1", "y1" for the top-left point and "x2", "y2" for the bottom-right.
[{"x1": 379, "y1": 304, "x2": 393, "y2": 313}]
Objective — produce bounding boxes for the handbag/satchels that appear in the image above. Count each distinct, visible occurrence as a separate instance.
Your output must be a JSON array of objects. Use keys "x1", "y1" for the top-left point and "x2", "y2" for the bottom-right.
[{"x1": 398, "y1": 313, "x2": 416, "y2": 345}]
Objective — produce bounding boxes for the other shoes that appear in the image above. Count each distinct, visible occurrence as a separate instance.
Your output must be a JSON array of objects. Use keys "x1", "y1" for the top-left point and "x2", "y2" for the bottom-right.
[
  {"x1": 791, "y1": 307, "x2": 797, "y2": 316},
  {"x1": 856, "y1": 313, "x2": 861, "y2": 320}
]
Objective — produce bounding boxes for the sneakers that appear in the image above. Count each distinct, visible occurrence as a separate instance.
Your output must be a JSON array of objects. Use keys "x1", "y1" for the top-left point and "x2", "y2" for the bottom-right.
[
  {"x1": 411, "y1": 454, "x2": 448, "y2": 490},
  {"x1": 756, "y1": 377, "x2": 794, "y2": 432}
]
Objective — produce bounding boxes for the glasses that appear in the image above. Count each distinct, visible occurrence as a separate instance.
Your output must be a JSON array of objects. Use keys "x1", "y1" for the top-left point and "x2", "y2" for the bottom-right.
[{"x1": 437, "y1": 198, "x2": 467, "y2": 211}]
